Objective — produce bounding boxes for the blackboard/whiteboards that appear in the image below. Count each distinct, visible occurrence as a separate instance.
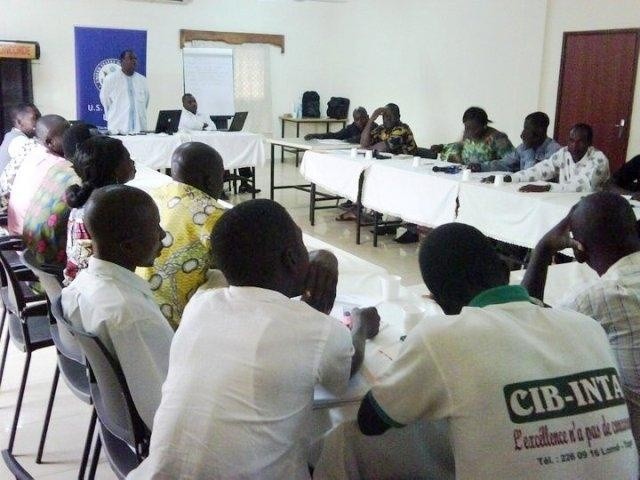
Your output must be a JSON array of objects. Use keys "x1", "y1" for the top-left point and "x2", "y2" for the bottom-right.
[{"x1": 183, "y1": 47, "x2": 234, "y2": 128}]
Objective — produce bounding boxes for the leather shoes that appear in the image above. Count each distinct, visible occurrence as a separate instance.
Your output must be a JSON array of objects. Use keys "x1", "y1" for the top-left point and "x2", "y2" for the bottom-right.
[
  {"x1": 375, "y1": 226, "x2": 396, "y2": 236},
  {"x1": 239, "y1": 185, "x2": 261, "y2": 193},
  {"x1": 394, "y1": 230, "x2": 419, "y2": 243}
]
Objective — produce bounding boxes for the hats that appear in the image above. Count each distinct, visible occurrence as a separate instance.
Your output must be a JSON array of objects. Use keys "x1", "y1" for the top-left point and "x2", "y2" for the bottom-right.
[{"x1": 463, "y1": 107, "x2": 492, "y2": 124}]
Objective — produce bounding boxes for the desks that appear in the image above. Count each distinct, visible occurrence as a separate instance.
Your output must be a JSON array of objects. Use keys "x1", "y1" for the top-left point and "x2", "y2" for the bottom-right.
[
  {"x1": 108, "y1": 130, "x2": 264, "y2": 200},
  {"x1": 279, "y1": 116, "x2": 348, "y2": 167},
  {"x1": 210, "y1": 115, "x2": 234, "y2": 129}
]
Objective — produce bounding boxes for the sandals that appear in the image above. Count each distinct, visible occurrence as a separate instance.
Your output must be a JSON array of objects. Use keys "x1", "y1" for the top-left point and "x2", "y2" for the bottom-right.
[{"x1": 335, "y1": 211, "x2": 357, "y2": 221}]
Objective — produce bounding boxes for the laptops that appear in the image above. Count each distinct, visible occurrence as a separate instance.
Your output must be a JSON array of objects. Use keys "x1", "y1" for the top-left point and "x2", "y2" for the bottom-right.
[
  {"x1": 139, "y1": 109, "x2": 183, "y2": 133},
  {"x1": 212, "y1": 112, "x2": 248, "y2": 132}
]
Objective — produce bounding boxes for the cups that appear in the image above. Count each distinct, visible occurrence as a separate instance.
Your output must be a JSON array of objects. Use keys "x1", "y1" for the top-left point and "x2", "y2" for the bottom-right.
[
  {"x1": 381, "y1": 274, "x2": 402, "y2": 301},
  {"x1": 495, "y1": 175, "x2": 504, "y2": 186},
  {"x1": 413, "y1": 157, "x2": 421, "y2": 167},
  {"x1": 366, "y1": 150, "x2": 373, "y2": 160},
  {"x1": 402, "y1": 308, "x2": 426, "y2": 333},
  {"x1": 463, "y1": 169, "x2": 471, "y2": 181},
  {"x1": 351, "y1": 148, "x2": 358, "y2": 158}
]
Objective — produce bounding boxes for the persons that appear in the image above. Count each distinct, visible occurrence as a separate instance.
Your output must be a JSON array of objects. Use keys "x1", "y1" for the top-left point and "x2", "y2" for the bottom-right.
[
  {"x1": 135, "y1": 137, "x2": 241, "y2": 337},
  {"x1": 178, "y1": 93, "x2": 262, "y2": 200},
  {"x1": 94, "y1": 46, "x2": 152, "y2": 134},
  {"x1": 304, "y1": 103, "x2": 420, "y2": 244},
  {"x1": 357, "y1": 221, "x2": 638, "y2": 477},
  {"x1": 123, "y1": 198, "x2": 381, "y2": 480},
  {"x1": 518, "y1": 190, "x2": 639, "y2": 446},
  {"x1": 0, "y1": 89, "x2": 138, "y2": 288},
  {"x1": 59, "y1": 186, "x2": 180, "y2": 459},
  {"x1": 429, "y1": 107, "x2": 640, "y2": 270}
]
